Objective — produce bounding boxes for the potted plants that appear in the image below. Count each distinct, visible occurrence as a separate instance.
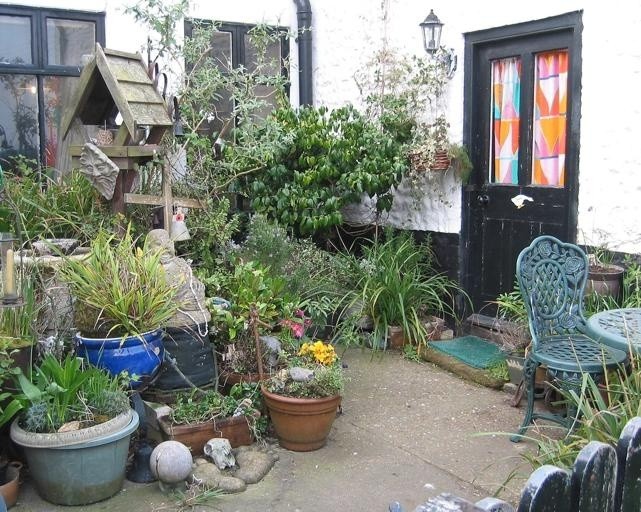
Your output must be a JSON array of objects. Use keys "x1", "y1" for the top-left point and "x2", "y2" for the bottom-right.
[
  {"x1": 320, "y1": 222, "x2": 477, "y2": 367},
  {"x1": 490, "y1": 291, "x2": 555, "y2": 386},
  {"x1": 574, "y1": 229, "x2": 629, "y2": 310}
]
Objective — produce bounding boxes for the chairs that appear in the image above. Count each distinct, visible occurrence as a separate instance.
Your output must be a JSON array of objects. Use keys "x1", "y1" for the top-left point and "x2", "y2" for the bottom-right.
[{"x1": 510, "y1": 236, "x2": 631, "y2": 445}]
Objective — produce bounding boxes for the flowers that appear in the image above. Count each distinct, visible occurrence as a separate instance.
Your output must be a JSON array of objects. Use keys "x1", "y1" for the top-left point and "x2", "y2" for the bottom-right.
[{"x1": 267, "y1": 303, "x2": 345, "y2": 402}]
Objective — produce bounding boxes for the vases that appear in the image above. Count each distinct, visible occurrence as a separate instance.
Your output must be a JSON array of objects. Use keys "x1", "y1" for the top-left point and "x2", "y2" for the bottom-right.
[{"x1": 259, "y1": 378, "x2": 344, "y2": 453}]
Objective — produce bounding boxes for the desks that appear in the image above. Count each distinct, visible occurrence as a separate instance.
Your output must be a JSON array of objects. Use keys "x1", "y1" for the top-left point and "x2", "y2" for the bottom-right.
[{"x1": 585, "y1": 305, "x2": 641, "y2": 353}]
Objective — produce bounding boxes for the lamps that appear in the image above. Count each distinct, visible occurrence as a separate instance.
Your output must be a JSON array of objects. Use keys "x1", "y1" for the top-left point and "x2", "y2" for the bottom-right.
[{"x1": 415, "y1": 10, "x2": 460, "y2": 78}]
